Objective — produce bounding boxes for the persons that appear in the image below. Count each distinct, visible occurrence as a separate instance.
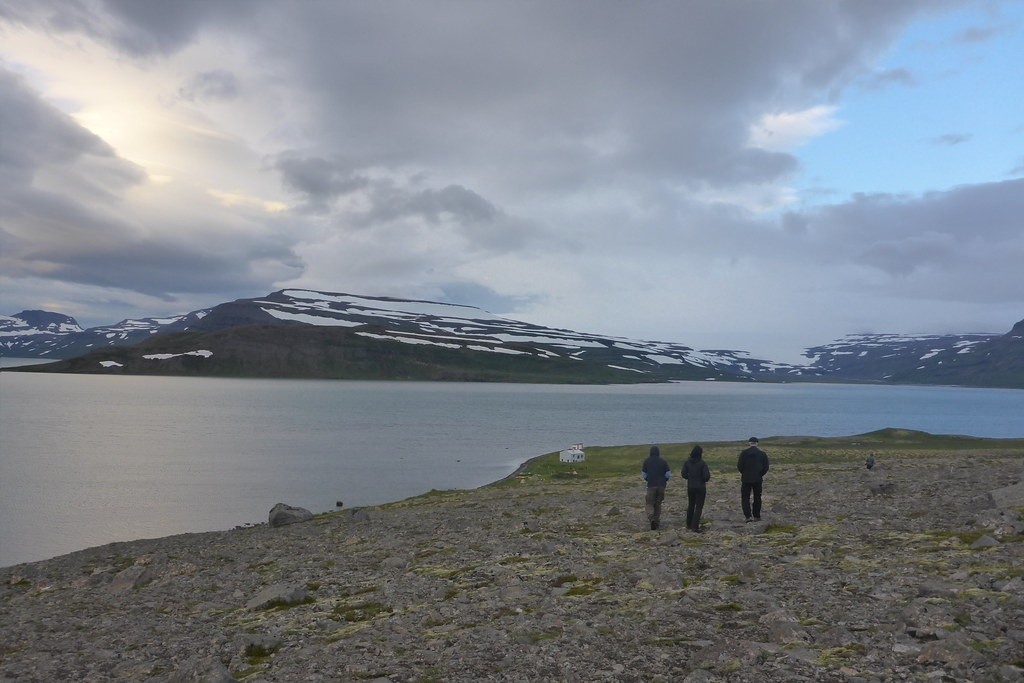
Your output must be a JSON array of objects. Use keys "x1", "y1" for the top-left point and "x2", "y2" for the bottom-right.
[
  {"x1": 681, "y1": 445, "x2": 711, "y2": 533},
  {"x1": 641, "y1": 446, "x2": 671, "y2": 530},
  {"x1": 737, "y1": 437, "x2": 769, "y2": 523},
  {"x1": 866, "y1": 453, "x2": 875, "y2": 469}
]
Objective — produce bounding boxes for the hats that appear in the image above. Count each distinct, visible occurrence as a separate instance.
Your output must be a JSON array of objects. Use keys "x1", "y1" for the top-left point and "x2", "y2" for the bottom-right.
[{"x1": 748, "y1": 436, "x2": 758, "y2": 442}]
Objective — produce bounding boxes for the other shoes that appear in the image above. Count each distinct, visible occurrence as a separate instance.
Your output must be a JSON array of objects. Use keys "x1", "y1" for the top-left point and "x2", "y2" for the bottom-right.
[
  {"x1": 651, "y1": 521, "x2": 656, "y2": 530},
  {"x1": 754, "y1": 517, "x2": 761, "y2": 521},
  {"x1": 693, "y1": 528, "x2": 702, "y2": 532},
  {"x1": 745, "y1": 518, "x2": 750, "y2": 522},
  {"x1": 686, "y1": 526, "x2": 691, "y2": 528}
]
